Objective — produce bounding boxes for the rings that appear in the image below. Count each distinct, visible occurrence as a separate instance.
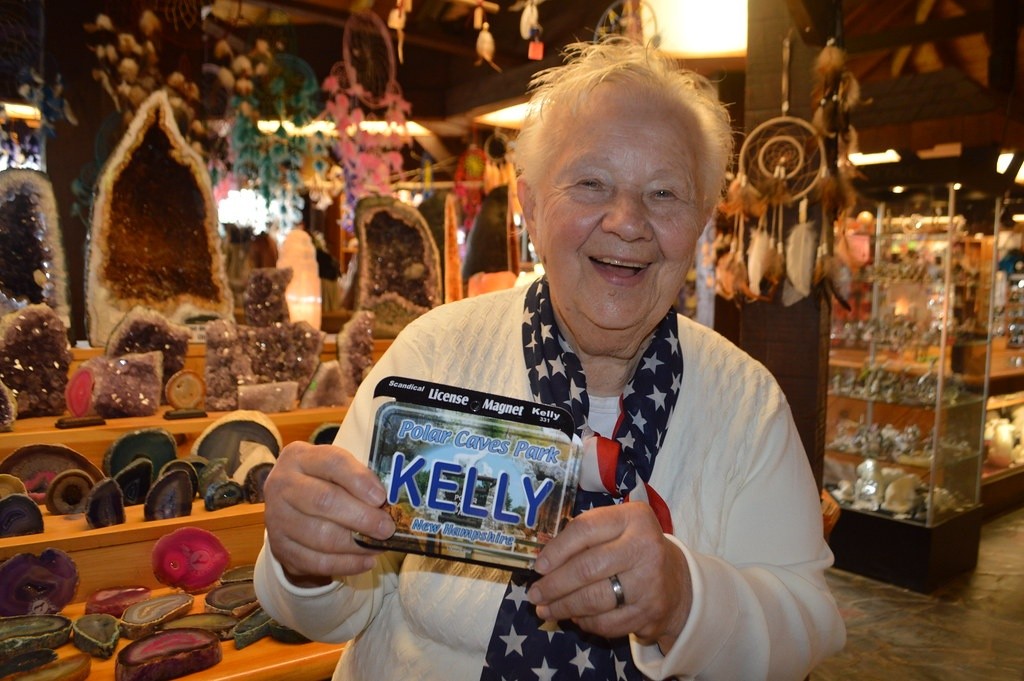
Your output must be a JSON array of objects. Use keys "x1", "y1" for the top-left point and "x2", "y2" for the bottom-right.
[{"x1": 608, "y1": 573, "x2": 626, "y2": 609}]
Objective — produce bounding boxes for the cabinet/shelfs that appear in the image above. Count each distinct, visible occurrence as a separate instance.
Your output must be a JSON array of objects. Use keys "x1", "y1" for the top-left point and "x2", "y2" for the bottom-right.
[
  {"x1": 0, "y1": 404, "x2": 351, "y2": 681},
  {"x1": 822, "y1": 158, "x2": 1024, "y2": 594}
]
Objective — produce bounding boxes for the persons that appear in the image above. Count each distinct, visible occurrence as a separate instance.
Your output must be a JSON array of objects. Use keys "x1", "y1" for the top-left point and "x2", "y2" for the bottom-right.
[{"x1": 254, "y1": 43, "x2": 846, "y2": 681}]
